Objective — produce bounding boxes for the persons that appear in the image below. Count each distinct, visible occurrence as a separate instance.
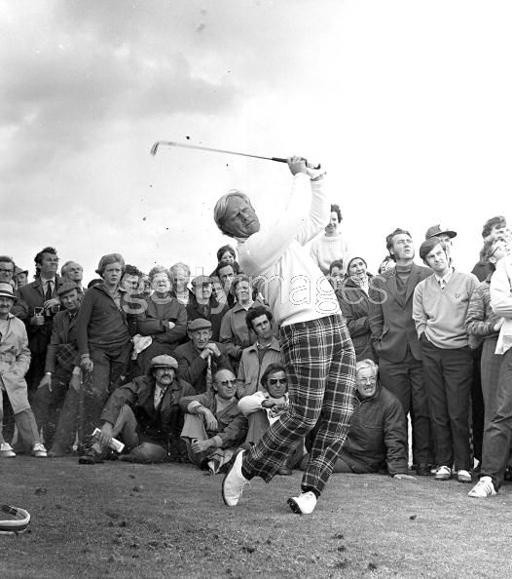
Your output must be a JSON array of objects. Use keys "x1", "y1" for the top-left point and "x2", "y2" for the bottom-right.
[
  {"x1": 312, "y1": 203, "x2": 512, "y2": 497},
  {"x1": 214, "y1": 156, "x2": 357, "y2": 512},
  {"x1": 0, "y1": 242, "x2": 306, "y2": 474}
]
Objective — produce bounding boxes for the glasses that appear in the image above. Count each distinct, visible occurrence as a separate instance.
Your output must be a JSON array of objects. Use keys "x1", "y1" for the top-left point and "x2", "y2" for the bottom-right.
[
  {"x1": 491, "y1": 245, "x2": 511, "y2": 257},
  {"x1": 266, "y1": 378, "x2": 288, "y2": 385},
  {"x1": 217, "y1": 379, "x2": 236, "y2": 386}
]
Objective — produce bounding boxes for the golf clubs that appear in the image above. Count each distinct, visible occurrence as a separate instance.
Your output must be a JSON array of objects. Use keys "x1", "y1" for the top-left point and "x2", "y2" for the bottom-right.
[{"x1": 151, "y1": 141, "x2": 321, "y2": 169}]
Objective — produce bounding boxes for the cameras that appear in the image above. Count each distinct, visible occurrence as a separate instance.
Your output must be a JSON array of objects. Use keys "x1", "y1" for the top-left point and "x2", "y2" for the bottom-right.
[{"x1": 46, "y1": 305, "x2": 61, "y2": 317}]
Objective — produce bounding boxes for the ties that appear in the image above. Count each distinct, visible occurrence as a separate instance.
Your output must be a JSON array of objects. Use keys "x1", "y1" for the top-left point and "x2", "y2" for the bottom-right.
[
  {"x1": 69, "y1": 313, "x2": 75, "y2": 322},
  {"x1": 45, "y1": 280, "x2": 53, "y2": 301},
  {"x1": 439, "y1": 277, "x2": 445, "y2": 291}
]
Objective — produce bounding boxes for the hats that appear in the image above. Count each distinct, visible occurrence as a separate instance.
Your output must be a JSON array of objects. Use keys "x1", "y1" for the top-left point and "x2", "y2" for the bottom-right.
[
  {"x1": 425, "y1": 223, "x2": 458, "y2": 241},
  {"x1": 13, "y1": 265, "x2": 29, "y2": 278},
  {"x1": 56, "y1": 279, "x2": 81, "y2": 296},
  {"x1": 187, "y1": 317, "x2": 213, "y2": 332},
  {"x1": 0, "y1": 282, "x2": 19, "y2": 300},
  {"x1": 191, "y1": 274, "x2": 213, "y2": 287},
  {"x1": 343, "y1": 252, "x2": 368, "y2": 276},
  {"x1": 150, "y1": 352, "x2": 179, "y2": 370}
]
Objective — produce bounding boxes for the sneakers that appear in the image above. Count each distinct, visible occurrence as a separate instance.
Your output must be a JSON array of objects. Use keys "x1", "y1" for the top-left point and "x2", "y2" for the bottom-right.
[
  {"x1": 286, "y1": 490, "x2": 318, "y2": 515},
  {"x1": 434, "y1": 465, "x2": 452, "y2": 480},
  {"x1": 0, "y1": 442, "x2": 17, "y2": 458},
  {"x1": 456, "y1": 469, "x2": 472, "y2": 483},
  {"x1": 31, "y1": 441, "x2": 49, "y2": 458},
  {"x1": 47, "y1": 443, "x2": 67, "y2": 458},
  {"x1": 411, "y1": 463, "x2": 437, "y2": 477},
  {"x1": 467, "y1": 475, "x2": 499, "y2": 498},
  {"x1": 77, "y1": 445, "x2": 106, "y2": 465},
  {"x1": 221, "y1": 447, "x2": 253, "y2": 508},
  {"x1": 277, "y1": 464, "x2": 292, "y2": 476}
]
